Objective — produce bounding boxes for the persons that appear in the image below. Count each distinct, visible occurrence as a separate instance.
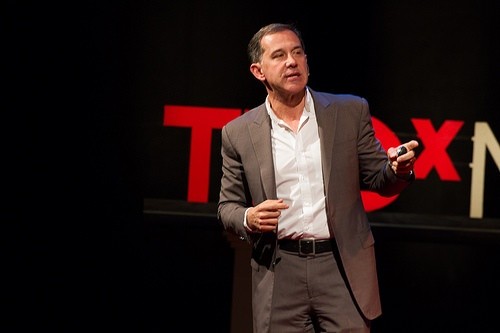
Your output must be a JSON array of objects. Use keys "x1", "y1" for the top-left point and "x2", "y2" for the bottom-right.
[{"x1": 217, "y1": 24, "x2": 419, "y2": 333}]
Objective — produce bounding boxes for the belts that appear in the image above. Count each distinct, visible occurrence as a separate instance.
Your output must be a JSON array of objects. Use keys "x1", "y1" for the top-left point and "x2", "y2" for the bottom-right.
[{"x1": 275, "y1": 239, "x2": 337, "y2": 255}]
